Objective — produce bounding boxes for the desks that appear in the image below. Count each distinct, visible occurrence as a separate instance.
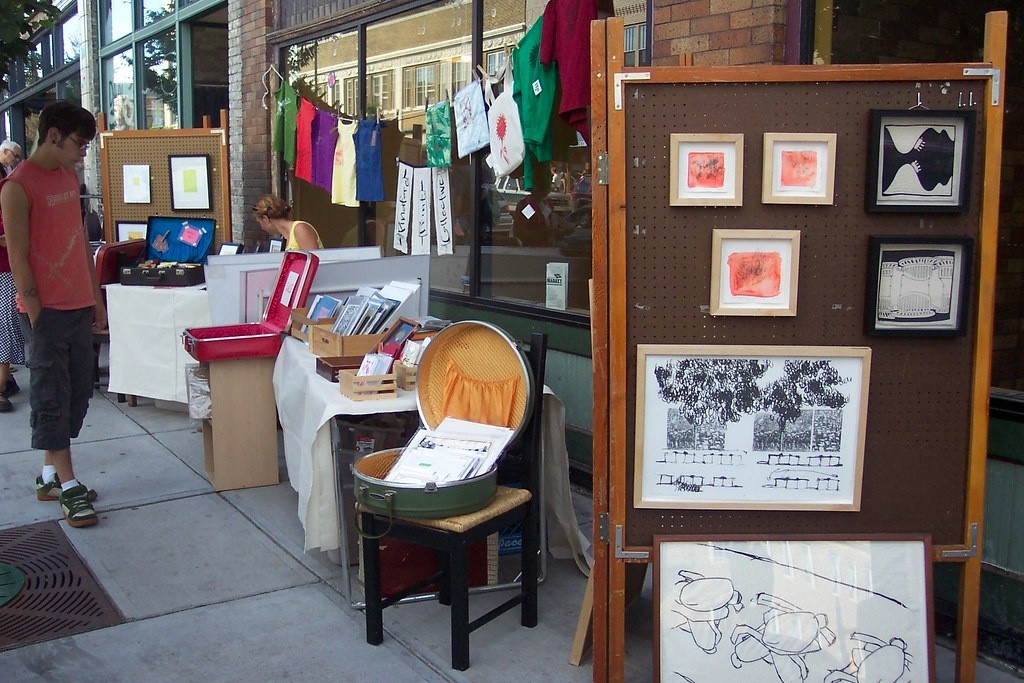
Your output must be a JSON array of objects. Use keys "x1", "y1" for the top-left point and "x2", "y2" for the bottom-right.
[
  {"x1": 100, "y1": 281, "x2": 211, "y2": 406},
  {"x1": 273, "y1": 333, "x2": 597, "y2": 609}
]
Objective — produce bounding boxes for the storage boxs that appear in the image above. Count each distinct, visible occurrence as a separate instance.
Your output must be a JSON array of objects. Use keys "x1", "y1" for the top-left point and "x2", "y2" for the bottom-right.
[{"x1": 184, "y1": 246, "x2": 523, "y2": 595}]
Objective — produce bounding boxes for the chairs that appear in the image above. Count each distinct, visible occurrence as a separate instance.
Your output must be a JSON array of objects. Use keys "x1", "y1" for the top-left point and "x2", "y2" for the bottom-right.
[
  {"x1": 353, "y1": 331, "x2": 549, "y2": 671},
  {"x1": 91, "y1": 239, "x2": 149, "y2": 408}
]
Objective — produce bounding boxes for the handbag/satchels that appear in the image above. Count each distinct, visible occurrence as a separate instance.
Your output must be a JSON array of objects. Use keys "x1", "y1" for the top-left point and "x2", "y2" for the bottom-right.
[{"x1": 485, "y1": 56, "x2": 525, "y2": 178}]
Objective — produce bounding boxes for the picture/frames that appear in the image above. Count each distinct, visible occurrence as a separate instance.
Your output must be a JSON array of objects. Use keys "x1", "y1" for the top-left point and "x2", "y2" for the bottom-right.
[
  {"x1": 669, "y1": 132, "x2": 744, "y2": 207},
  {"x1": 863, "y1": 235, "x2": 975, "y2": 338},
  {"x1": 115, "y1": 220, "x2": 149, "y2": 243},
  {"x1": 369, "y1": 316, "x2": 422, "y2": 359},
  {"x1": 120, "y1": 163, "x2": 153, "y2": 203},
  {"x1": 633, "y1": 344, "x2": 873, "y2": 512},
  {"x1": 652, "y1": 533, "x2": 938, "y2": 683},
  {"x1": 865, "y1": 108, "x2": 977, "y2": 214},
  {"x1": 761, "y1": 133, "x2": 837, "y2": 207},
  {"x1": 710, "y1": 228, "x2": 801, "y2": 316},
  {"x1": 239, "y1": 253, "x2": 431, "y2": 325},
  {"x1": 167, "y1": 153, "x2": 213, "y2": 212}
]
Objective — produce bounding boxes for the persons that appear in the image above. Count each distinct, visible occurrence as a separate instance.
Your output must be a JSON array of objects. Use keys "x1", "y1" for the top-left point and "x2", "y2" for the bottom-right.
[
  {"x1": 0, "y1": 102, "x2": 108, "y2": 528},
  {"x1": 252, "y1": 194, "x2": 326, "y2": 251}
]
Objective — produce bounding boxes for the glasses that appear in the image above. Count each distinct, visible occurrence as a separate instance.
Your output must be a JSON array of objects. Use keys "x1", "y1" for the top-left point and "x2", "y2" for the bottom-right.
[
  {"x1": 66, "y1": 136, "x2": 90, "y2": 151},
  {"x1": 8, "y1": 148, "x2": 19, "y2": 160}
]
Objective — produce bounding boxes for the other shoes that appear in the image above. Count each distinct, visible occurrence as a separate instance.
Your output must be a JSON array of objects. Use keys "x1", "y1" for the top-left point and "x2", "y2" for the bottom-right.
[
  {"x1": 0, "y1": 374, "x2": 20, "y2": 397},
  {"x1": 0, "y1": 394, "x2": 13, "y2": 411}
]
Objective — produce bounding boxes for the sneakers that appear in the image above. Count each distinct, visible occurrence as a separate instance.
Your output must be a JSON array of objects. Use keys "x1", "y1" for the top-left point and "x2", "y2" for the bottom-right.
[
  {"x1": 60, "y1": 479, "x2": 98, "y2": 526},
  {"x1": 36, "y1": 472, "x2": 97, "y2": 500}
]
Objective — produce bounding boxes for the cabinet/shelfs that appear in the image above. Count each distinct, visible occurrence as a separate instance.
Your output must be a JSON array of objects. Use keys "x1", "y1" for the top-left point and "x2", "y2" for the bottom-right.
[{"x1": 199, "y1": 355, "x2": 280, "y2": 492}]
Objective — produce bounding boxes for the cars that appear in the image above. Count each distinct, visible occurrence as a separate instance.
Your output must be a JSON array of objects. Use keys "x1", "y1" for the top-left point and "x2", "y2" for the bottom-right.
[{"x1": 453, "y1": 165, "x2": 593, "y2": 279}]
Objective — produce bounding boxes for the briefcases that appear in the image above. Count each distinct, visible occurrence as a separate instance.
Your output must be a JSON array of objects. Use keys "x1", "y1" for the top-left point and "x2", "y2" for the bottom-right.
[
  {"x1": 120, "y1": 215, "x2": 216, "y2": 286},
  {"x1": 349, "y1": 319, "x2": 536, "y2": 539},
  {"x1": 180, "y1": 247, "x2": 319, "y2": 362}
]
